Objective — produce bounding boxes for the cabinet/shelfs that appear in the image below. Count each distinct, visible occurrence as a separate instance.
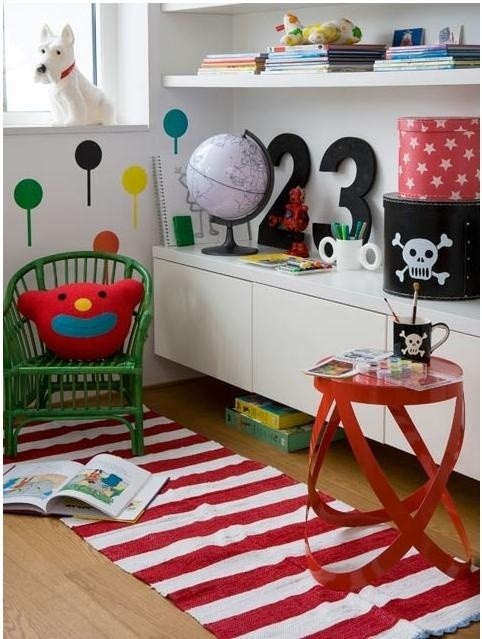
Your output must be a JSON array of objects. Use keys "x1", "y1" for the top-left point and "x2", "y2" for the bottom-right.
[
  {"x1": 150, "y1": 240, "x2": 480, "y2": 482},
  {"x1": 161, "y1": 3, "x2": 480, "y2": 89}
]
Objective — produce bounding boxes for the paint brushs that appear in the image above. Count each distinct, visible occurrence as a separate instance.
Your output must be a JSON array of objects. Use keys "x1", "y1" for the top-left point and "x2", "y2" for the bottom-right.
[{"x1": 384, "y1": 281, "x2": 420, "y2": 326}]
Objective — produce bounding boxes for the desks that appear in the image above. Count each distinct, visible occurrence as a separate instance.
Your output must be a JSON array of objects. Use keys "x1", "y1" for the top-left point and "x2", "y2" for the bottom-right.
[{"x1": 303, "y1": 353, "x2": 473, "y2": 592}]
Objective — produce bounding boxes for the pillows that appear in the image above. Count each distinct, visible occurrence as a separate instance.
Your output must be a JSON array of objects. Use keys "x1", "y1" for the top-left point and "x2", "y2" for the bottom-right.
[{"x1": 17, "y1": 279, "x2": 144, "y2": 360}]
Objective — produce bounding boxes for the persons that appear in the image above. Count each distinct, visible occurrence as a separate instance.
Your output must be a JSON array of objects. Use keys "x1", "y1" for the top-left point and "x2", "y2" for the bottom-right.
[{"x1": 268, "y1": 185, "x2": 310, "y2": 259}]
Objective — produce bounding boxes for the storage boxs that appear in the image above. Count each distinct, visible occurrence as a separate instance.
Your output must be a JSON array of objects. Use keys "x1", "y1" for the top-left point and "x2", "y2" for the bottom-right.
[
  {"x1": 398, "y1": 116, "x2": 480, "y2": 201},
  {"x1": 383, "y1": 192, "x2": 480, "y2": 301}
]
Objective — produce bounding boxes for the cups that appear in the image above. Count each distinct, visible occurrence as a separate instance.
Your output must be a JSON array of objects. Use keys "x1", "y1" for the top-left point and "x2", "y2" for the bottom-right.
[
  {"x1": 392, "y1": 315, "x2": 449, "y2": 367},
  {"x1": 318, "y1": 236, "x2": 381, "y2": 273}
]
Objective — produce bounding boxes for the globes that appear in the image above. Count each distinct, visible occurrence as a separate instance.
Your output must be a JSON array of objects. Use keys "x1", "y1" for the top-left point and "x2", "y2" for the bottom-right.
[{"x1": 186, "y1": 128, "x2": 275, "y2": 257}]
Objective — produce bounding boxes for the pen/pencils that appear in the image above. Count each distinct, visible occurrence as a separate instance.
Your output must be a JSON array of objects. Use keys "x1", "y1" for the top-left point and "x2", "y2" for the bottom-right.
[
  {"x1": 278, "y1": 259, "x2": 333, "y2": 272},
  {"x1": 331, "y1": 221, "x2": 367, "y2": 239}
]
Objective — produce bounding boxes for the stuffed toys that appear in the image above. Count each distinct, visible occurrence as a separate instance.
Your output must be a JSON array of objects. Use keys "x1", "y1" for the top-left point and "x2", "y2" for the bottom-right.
[
  {"x1": 31, "y1": 22, "x2": 116, "y2": 128},
  {"x1": 275, "y1": 11, "x2": 365, "y2": 44}
]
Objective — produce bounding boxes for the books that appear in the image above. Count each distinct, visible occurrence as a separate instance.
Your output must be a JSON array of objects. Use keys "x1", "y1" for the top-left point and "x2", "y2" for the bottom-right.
[
  {"x1": 191, "y1": 25, "x2": 480, "y2": 71},
  {"x1": 1, "y1": 452, "x2": 170, "y2": 525}
]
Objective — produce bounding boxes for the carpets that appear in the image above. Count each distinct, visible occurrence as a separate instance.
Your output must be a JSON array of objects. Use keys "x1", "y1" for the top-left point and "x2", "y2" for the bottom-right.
[{"x1": 3, "y1": 402, "x2": 480, "y2": 639}]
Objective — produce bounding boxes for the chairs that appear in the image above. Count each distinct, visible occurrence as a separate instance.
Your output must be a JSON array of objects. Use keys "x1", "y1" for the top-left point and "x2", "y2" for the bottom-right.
[{"x1": 4, "y1": 251, "x2": 155, "y2": 456}]
